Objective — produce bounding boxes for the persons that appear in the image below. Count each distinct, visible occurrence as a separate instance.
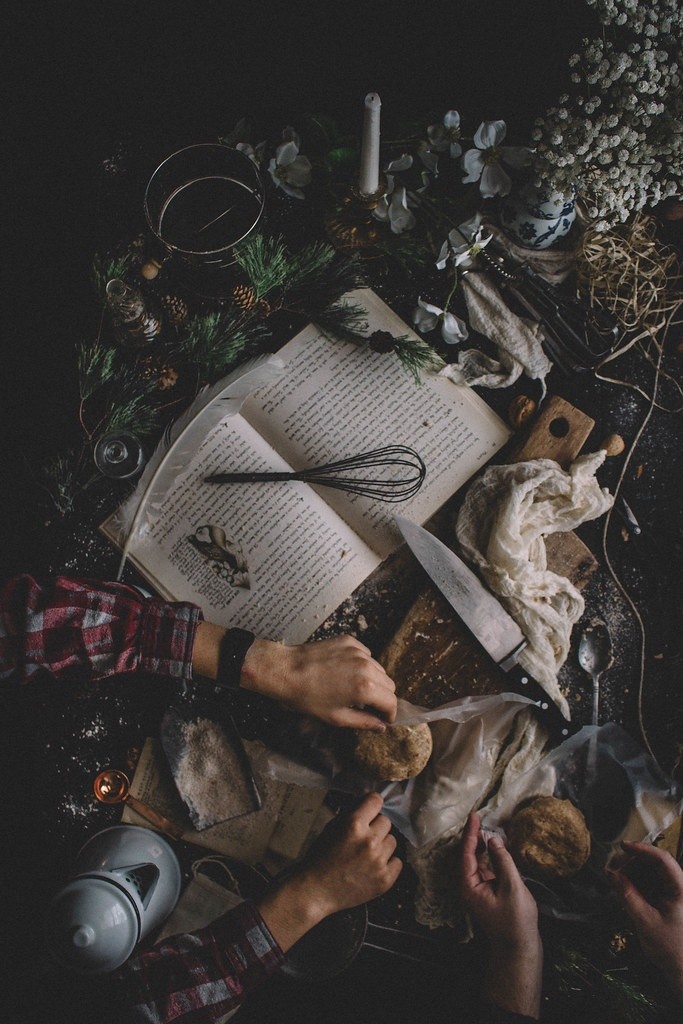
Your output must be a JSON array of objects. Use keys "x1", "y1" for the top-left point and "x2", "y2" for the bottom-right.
[
  {"x1": 0, "y1": 575, "x2": 404, "y2": 1024},
  {"x1": 458, "y1": 813, "x2": 683, "y2": 1023}
]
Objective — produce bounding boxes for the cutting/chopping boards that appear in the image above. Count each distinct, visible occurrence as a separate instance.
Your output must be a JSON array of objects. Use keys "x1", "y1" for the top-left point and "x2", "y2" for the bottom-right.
[{"x1": 338, "y1": 394, "x2": 601, "y2": 759}]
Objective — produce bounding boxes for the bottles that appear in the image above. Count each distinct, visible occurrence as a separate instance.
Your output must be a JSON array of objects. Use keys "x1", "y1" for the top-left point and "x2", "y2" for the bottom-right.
[
  {"x1": 94, "y1": 432, "x2": 147, "y2": 480},
  {"x1": 106, "y1": 278, "x2": 163, "y2": 346}
]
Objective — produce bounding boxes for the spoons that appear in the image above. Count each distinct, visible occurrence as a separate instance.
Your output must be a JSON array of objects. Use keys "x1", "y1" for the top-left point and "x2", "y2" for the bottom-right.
[
  {"x1": 577, "y1": 620, "x2": 614, "y2": 775},
  {"x1": 94, "y1": 769, "x2": 182, "y2": 840}
]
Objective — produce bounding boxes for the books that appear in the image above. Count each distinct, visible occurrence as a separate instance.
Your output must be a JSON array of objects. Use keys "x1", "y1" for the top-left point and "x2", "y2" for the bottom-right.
[{"x1": 99, "y1": 282, "x2": 514, "y2": 646}]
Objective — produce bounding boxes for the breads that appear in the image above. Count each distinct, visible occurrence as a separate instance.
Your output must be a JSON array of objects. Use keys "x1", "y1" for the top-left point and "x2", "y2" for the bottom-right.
[
  {"x1": 353, "y1": 724, "x2": 432, "y2": 780},
  {"x1": 509, "y1": 797, "x2": 592, "y2": 877}
]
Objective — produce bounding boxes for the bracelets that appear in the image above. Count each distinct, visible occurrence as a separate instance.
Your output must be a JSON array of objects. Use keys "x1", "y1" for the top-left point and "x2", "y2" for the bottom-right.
[{"x1": 216, "y1": 627, "x2": 255, "y2": 691}]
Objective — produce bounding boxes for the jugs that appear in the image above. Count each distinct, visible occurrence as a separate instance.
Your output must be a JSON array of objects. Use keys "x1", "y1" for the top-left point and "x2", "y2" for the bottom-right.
[{"x1": 43, "y1": 825, "x2": 181, "y2": 979}]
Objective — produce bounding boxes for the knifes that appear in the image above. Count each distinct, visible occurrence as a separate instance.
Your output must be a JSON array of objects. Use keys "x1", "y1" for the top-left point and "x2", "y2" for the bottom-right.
[{"x1": 390, "y1": 513, "x2": 584, "y2": 747}]
